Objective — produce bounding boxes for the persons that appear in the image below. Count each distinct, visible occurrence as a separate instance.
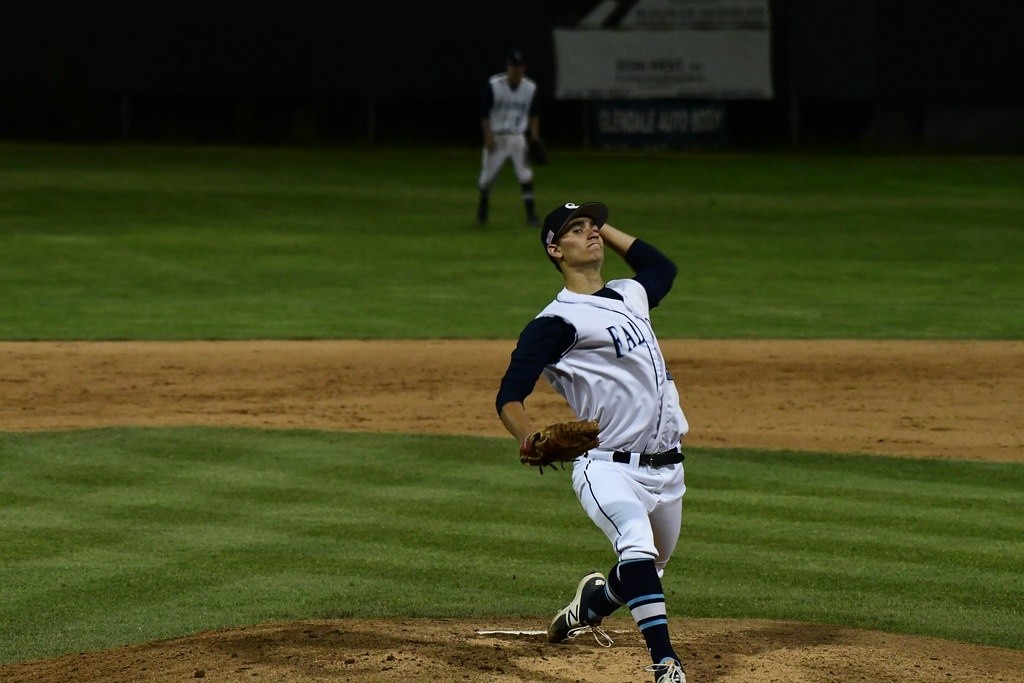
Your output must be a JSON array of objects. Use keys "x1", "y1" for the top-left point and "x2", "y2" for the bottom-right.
[
  {"x1": 496, "y1": 201, "x2": 689, "y2": 683},
  {"x1": 475, "y1": 50, "x2": 544, "y2": 225}
]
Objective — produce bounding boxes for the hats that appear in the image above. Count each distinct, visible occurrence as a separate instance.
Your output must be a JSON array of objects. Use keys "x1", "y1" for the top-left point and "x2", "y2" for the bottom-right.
[{"x1": 540, "y1": 200, "x2": 610, "y2": 257}]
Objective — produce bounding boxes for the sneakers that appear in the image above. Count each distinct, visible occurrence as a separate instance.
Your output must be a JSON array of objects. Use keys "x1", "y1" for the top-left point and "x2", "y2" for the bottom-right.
[
  {"x1": 646, "y1": 657, "x2": 686, "y2": 683},
  {"x1": 548, "y1": 572, "x2": 614, "y2": 648}
]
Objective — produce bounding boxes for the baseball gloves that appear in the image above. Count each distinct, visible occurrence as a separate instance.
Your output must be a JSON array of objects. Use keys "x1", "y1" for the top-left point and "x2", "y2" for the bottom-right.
[
  {"x1": 530, "y1": 138, "x2": 548, "y2": 166},
  {"x1": 520, "y1": 418, "x2": 600, "y2": 466}
]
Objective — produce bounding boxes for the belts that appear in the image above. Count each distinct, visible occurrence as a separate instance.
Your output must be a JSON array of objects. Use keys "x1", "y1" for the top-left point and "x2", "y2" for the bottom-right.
[{"x1": 584, "y1": 439, "x2": 686, "y2": 470}]
